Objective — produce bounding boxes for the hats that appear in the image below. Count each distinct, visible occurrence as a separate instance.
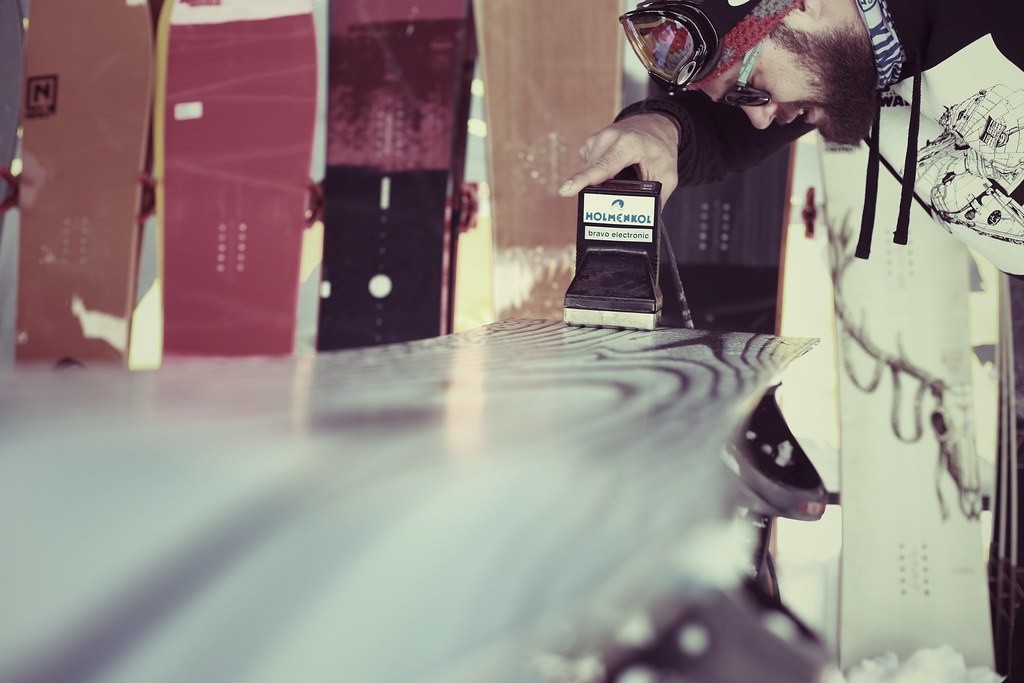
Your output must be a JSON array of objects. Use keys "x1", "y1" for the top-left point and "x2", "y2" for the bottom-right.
[{"x1": 618, "y1": 0, "x2": 804, "y2": 93}]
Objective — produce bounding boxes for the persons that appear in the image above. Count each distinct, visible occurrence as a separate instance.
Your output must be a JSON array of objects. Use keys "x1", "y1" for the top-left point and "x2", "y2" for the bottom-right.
[{"x1": 557, "y1": 0, "x2": 1023, "y2": 281}]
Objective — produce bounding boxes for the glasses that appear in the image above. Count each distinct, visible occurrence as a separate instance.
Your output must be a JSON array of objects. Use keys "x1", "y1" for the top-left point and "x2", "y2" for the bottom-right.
[
  {"x1": 619, "y1": 2, "x2": 724, "y2": 92},
  {"x1": 716, "y1": 38, "x2": 772, "y2": 110}
]
